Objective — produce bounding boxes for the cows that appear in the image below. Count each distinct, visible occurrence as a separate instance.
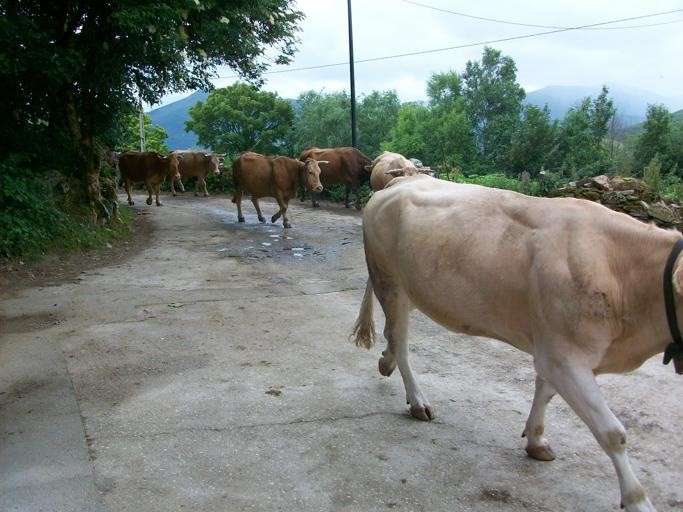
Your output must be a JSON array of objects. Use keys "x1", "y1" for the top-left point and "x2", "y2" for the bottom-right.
[
  {"x1": 116, "y1": 149, "x2": 182, "y2": 208},
  {"x1": 371, "y1": 150, "x2": 432, "y2": 191},
  {"x1": 298, "y1": 145, "x2": 376, "y2": 211},
  {"x1": 231, "y1": 151, "x2": 329, "y2": 228},
  {"x1": 166, "y1": 149, "x2": 230, "y2": 197},
  {"x1": 346, "y1": 170, "x2": 683, "y2": 511}
]
